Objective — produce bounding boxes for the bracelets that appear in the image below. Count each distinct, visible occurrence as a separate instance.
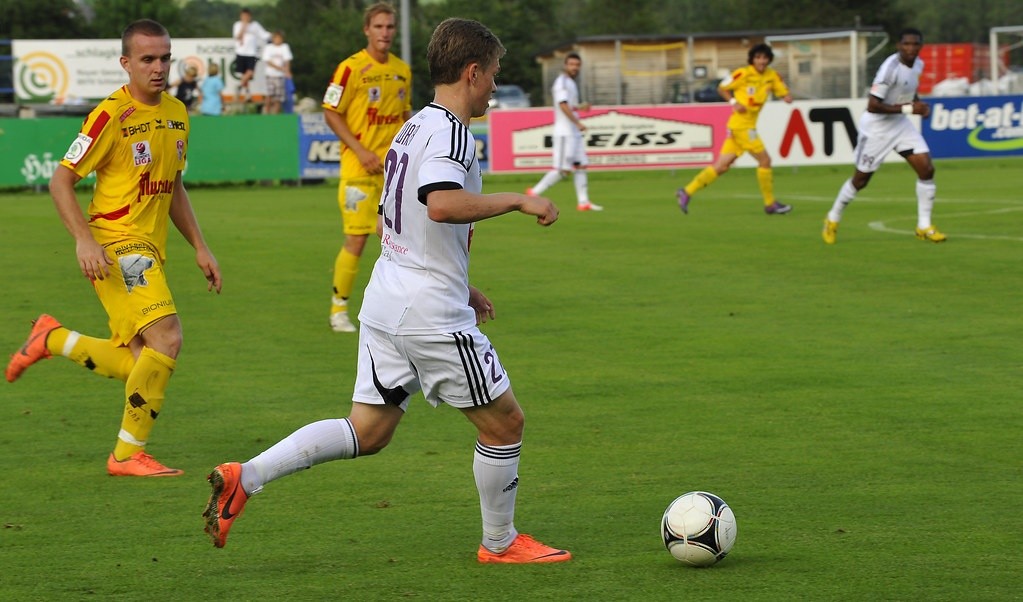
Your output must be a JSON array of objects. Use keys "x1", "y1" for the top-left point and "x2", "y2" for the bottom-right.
[
  {"x1": 729, "y1": 98, "x2": 738, "y2": 106},
  {"x1": 902, "y1": 104, "x2": 913, "y2": 115}
]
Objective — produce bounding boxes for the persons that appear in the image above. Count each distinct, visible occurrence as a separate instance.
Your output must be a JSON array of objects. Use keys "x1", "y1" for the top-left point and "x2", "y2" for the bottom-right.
[
  {"x1": 167, "y1": 9, "x2": 293, "y2": 116},
  {"x1": 675, "y1": 42, "x2": 793, "y2": 215},
  {"x1": 526, "y1": 53, "x2": 603, "y2": 211},
  {"x1": 321, "y1": 4, "x2": 414, "y2": 331},
  {"x1": 822, "y1": 29, "x2": 947, "y2": 245},
  {"x1": 203, "y1": 18, "x2": 571, "y2": 564},
  {"x1": 8, "y1": 19, "x2": 223, "y2": 474}
]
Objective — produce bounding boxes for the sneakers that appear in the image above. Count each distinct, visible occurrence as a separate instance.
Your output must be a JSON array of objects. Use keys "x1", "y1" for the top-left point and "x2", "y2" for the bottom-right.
[
  {"x1": 765, "y1": 201, "x2": 792, "y2": 214},
  {"x1": 202, "y1": 461, "x2": 253, "y2": 549},
  {"x1": 477, "y1": 534, "x2": 571, "y2": 565},
  {"x1": 106, "y1": 450, "x2": 185, "y2": 477},
  {"x1": 677, "y1": 188, "x2": 690, "y2": 214},
  {"x1": 575, "y1": 201, "x2": 603, "y2": 212},
  {"x1": 525, "y1": 187, "x2": 540, "y2": 198},
  {"x1": 328, "y1": 311, "x2": 357, "y2": 333},
  {"x1": 821, "y1": 216, "x2": 839, "y2": 245},
  {"x1": 915, "y1": 223, "x2": 948, "y2": 242},
  {"x1": 6, "y1": 312, "x2": 63, "y2": 383}
]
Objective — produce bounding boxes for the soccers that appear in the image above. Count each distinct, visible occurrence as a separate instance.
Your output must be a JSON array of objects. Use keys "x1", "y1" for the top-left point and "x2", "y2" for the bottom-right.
[{"x1": 661, "y1": 491, "x2": 737, "y2": 567}]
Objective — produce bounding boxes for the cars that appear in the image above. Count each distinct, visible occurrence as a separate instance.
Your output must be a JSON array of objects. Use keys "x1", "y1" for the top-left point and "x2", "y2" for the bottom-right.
[
  {"x1": 491, "y1": 86, "x2": 533, "y2": 109},
  {"x1": 679, "y1": 79, "x2": 734, "y2": 103}
]
